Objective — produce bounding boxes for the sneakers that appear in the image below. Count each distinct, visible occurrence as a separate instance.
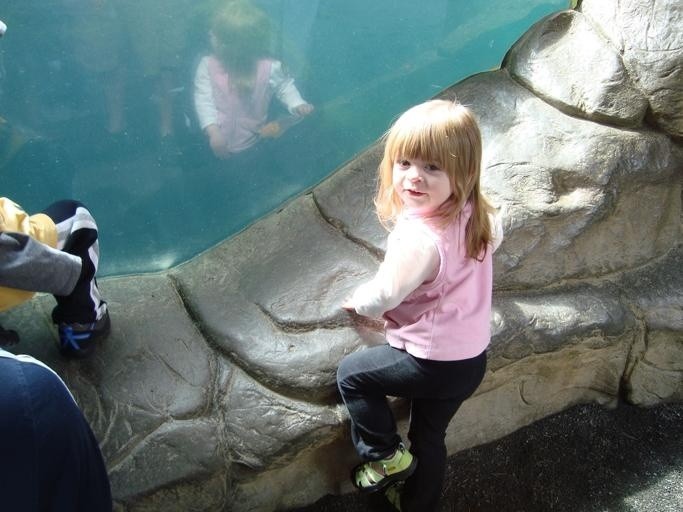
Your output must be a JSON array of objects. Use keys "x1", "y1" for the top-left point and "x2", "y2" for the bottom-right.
[
  {"x1": 351, "y1": 442, "x2": 418, "y2": 510},
  {"x1": 0, "y1": 326, "x2": 19, "y2": 351},
  {"x1": 58, "y1": 309, "x2": 110, "y2": 360}
]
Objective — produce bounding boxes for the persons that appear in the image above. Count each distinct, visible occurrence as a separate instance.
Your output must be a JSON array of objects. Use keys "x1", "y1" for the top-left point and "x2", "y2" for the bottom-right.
[
  {"x1": 334, "y1": 97, "x2": 506, "y2": 512},
  {"x1": 1, "y1": 346, "x2": 116, "y2": 510},
  {"x1": 1, "y1": 195, "x2": 112, "y2": 360}
]
